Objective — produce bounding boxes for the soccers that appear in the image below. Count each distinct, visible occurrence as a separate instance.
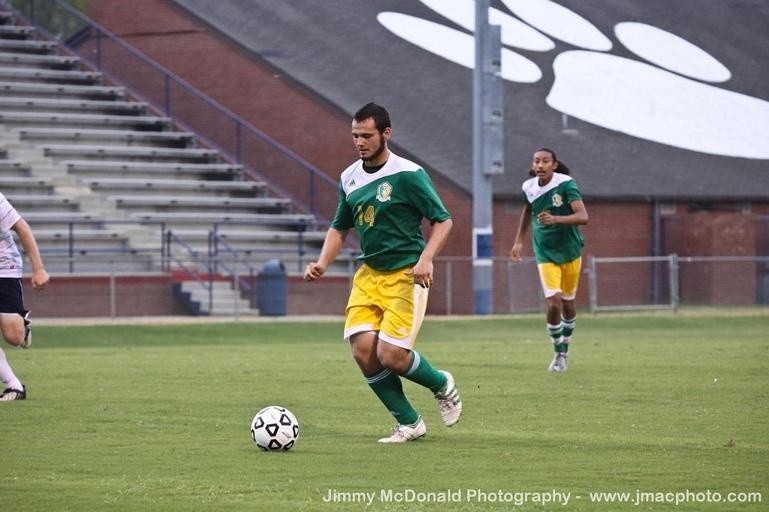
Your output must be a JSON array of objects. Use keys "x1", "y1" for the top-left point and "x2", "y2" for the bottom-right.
[{"x1": 252, "y1": 406, "x2": 299, "y2": 451}]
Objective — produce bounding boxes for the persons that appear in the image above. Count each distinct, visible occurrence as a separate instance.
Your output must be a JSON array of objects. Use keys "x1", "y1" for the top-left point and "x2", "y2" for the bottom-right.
[
  {"x1": 301, "y1": 100, "x2": 465, "y2": 443},
  {"x1": 509, "y1": 147, "x2": 592, "y2": 376},
  {"x1": 0, "y1": 190, "x2": 52, "y2": 403}
]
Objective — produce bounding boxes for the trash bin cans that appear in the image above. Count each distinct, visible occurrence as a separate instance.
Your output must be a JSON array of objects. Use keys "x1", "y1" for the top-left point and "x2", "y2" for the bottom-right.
[{"x1": 257, "y1": 259, "x2": 288, "y2": 316}]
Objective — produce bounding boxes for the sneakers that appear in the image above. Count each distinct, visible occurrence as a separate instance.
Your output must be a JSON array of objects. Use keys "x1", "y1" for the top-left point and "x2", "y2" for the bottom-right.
[
  {"x1": 549, "y1": 353, "x2": 568, "y2": 372},
  {"x1": 378, "y1": 415, "x2": 426, "y2": 443},
  {"x1": 434, "y1": 371, "x2": 462, "y2": 427},
  {"x1": 20, "y1": 327, "x2": 31, "y2": 348},
  {"x1": 0, "y1": 385, "x2": 26, "y2": 401}
]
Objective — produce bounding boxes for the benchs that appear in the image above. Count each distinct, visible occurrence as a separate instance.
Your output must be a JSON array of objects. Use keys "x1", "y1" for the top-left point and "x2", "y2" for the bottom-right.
[
  {"x1": 1, "y1": 98, "x2": 242, "y2": 175},
  {"x1": 1, "y1": 5, "x2": 127, "y2": 99}
]
